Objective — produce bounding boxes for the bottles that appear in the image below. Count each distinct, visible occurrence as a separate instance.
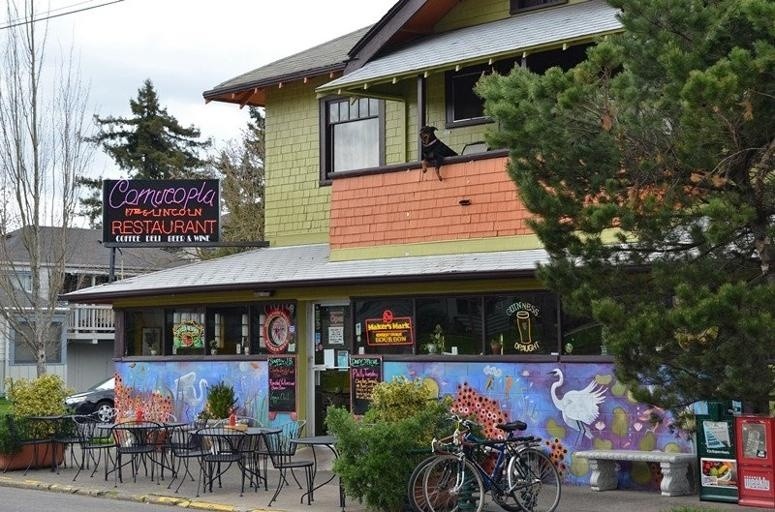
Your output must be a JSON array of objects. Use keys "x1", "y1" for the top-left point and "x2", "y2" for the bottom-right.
[
  {"x1": 229, "y1": 409, "x2": 235, "y2": 427},
  {"x1": 135, "y1": 410, "x2": 142, "y2": 424}
]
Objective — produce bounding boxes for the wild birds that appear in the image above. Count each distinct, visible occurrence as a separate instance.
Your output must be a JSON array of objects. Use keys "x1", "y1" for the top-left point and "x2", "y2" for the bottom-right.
[
  {"x1": 174, "y1": 371, "x2": 211, "y2": 423},
  {"x1": 546, "y1": 368, "x2": 608, "y2": 449}
]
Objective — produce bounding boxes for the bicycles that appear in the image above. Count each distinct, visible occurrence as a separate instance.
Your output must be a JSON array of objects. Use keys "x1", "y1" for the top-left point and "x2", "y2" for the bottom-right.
[{"x1": 405, "y1": 412, "x2": 561, "y2": 512}]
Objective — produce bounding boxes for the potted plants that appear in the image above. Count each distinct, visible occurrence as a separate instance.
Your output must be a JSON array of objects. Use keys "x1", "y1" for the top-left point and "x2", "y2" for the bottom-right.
[
  {"x1": 324, "y1": 393, "x2": 491, "y2": 511},
  {"x1": 194, "y1": 383, "x2": 248, "y2": 450},
  {"x1": 0, "y1": 375, "x2": 75, "y2": 473}
]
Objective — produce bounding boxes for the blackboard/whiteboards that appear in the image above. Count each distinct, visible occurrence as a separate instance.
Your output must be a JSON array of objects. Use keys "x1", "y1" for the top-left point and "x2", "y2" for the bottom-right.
[
  {"x1": 349, "y1": 354, "x2": 383, "y2": 418},
  {"x1": 267, "y1": 354, "x2": 296, "y2": 414}
]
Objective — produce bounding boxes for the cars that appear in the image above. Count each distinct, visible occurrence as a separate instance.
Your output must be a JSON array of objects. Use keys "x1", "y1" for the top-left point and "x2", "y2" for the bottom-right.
[{"x1": 63, "y1": 373, "x2": 117, "y2": 424}]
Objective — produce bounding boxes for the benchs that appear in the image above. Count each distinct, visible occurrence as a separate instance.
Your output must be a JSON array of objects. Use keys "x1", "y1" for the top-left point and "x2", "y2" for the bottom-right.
[{"x1": 572, "y1": 448, "x2": 697, "y2": 496}]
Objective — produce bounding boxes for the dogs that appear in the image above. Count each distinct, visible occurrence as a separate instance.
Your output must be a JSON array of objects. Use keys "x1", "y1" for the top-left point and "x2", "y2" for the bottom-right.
[{"x1": 419, "y1": 126, "x2": 458, "y2": 182}]
